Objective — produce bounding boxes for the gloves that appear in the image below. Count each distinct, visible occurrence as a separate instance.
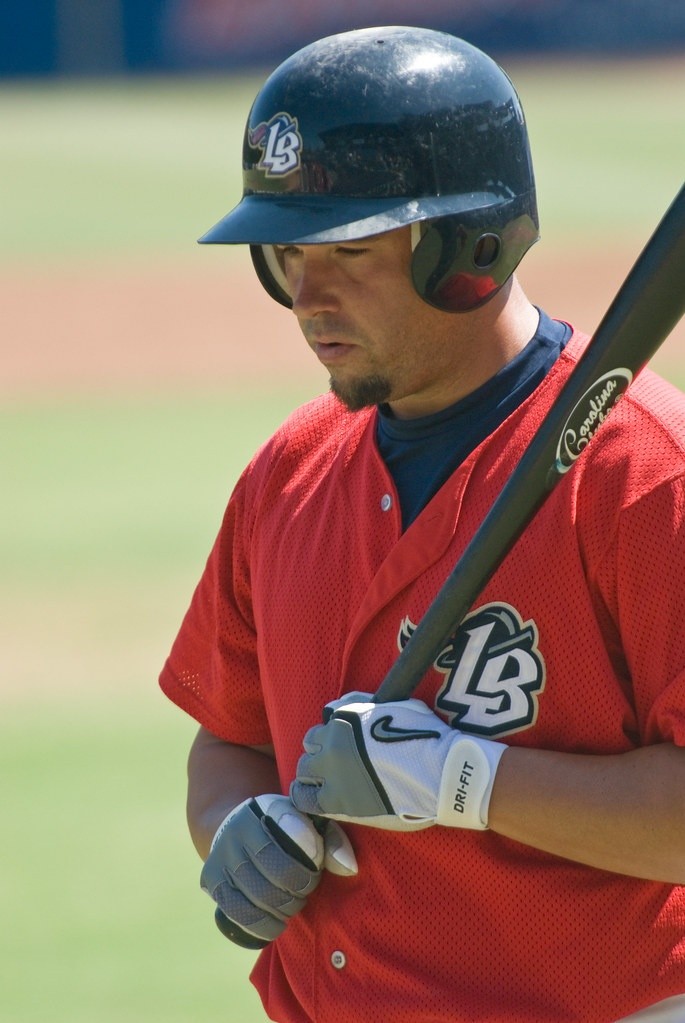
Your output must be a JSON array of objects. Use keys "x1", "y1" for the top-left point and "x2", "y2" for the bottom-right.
[
  {"x1": 197, "y1": 793, "x2": 358, "y2": 942},
  {"x1": 288, "y1": 692, "x2": 510, "y2": 833}
]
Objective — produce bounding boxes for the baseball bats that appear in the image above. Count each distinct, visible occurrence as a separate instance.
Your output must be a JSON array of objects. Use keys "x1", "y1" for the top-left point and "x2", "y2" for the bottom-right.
[{"x1": 213, "y1": 180, "x2": 684, "y2": 951}]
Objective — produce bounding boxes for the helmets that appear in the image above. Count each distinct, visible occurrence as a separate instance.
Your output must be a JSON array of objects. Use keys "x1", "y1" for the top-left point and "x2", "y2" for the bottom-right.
[{"x1": 195, "y1": 25, "x2": 539, "y2": 315}]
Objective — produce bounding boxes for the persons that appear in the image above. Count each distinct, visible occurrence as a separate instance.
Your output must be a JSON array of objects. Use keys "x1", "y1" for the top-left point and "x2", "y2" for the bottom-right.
[{"x1": 157, "y1": 27, "x2": 685, "y2": 1023}]
